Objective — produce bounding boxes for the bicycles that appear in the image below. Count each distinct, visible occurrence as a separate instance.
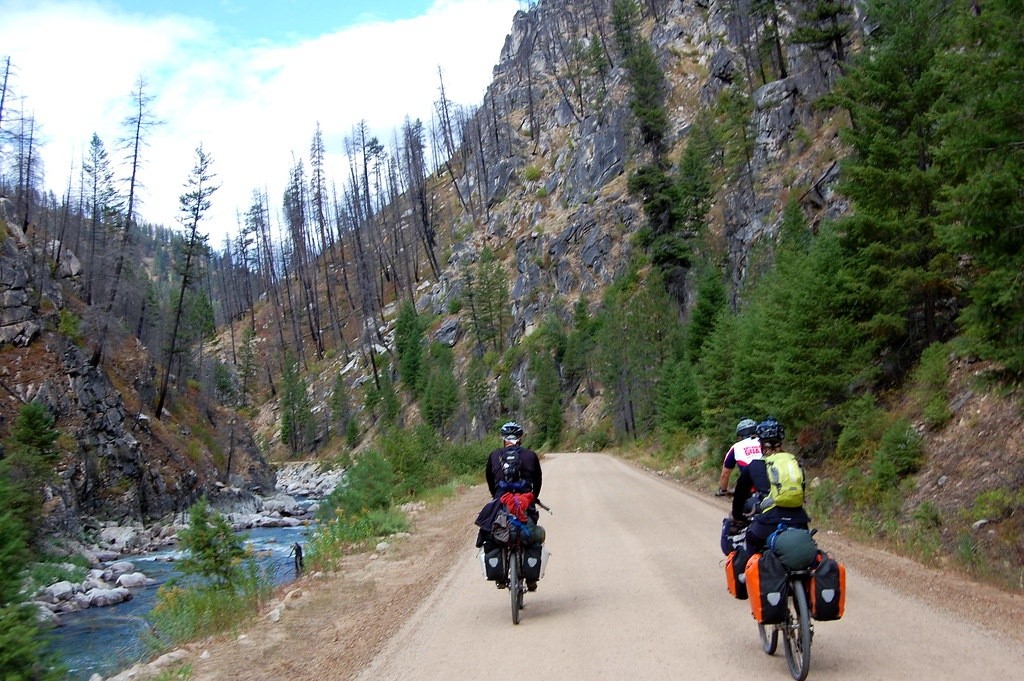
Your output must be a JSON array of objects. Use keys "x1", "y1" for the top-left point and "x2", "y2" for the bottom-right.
[
  {"x1": 716, "y1": 489, "x2": 815, "y2": 681},
  {"x1": 501, "y1": 498, "x2": 538, "y2": 625}
]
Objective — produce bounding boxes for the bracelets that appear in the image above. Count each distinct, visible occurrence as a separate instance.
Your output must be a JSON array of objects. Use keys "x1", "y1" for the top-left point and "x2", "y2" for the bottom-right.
[{"x1": 719, "y1": 488, "x2": 727, "y2": 493}]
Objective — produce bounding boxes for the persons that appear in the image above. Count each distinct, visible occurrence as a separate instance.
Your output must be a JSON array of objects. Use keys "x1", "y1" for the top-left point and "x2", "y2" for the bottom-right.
[
  {"x1": 484, "y1": 422, "x2": 542, "y2": 593},
  {"x1": 294, "y1": 543, "x2": 302, "y2": 572},
  {"x1": 718, "y1": 418, "x2": 814, "y2": 654}
]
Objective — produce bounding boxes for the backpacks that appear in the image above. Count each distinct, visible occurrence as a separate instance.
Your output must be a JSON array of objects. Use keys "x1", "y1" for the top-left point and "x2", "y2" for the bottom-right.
[
  {"x1": 497, "y1": 447, "x2": 523, "y2": 484},
  {"x1": 759, "y1": 451, "x2": 807, "y2": 514}
]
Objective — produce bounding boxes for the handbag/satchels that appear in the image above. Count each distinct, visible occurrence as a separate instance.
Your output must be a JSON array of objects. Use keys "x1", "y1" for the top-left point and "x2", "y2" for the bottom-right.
[
  {"x1": 724, "y1": 545, "x2": 753, "y2": 600},
  {"x1": 744, "y1": 549, "x2": 788, "y2": 625},
  {"x1": 807, "y1": 549, "x2": 847, "y2": 621}
]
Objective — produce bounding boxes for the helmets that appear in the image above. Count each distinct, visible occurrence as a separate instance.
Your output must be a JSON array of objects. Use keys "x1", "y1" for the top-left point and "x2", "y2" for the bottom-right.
[
  {"x1": 736, "y1": 417, "x2": 758, "y2": 433},
  {"x1": 501, "y1": 421, "x2": 523, "y2": 435},
  {"x1": 755, "y1": 417, "x2": 785, "y2": 439}
]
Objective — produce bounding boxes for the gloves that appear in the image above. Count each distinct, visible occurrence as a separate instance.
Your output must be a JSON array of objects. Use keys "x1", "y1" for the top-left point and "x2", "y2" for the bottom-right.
[{"x1": 715, "y1": 487, "x2": 727, "y2": 496}]
